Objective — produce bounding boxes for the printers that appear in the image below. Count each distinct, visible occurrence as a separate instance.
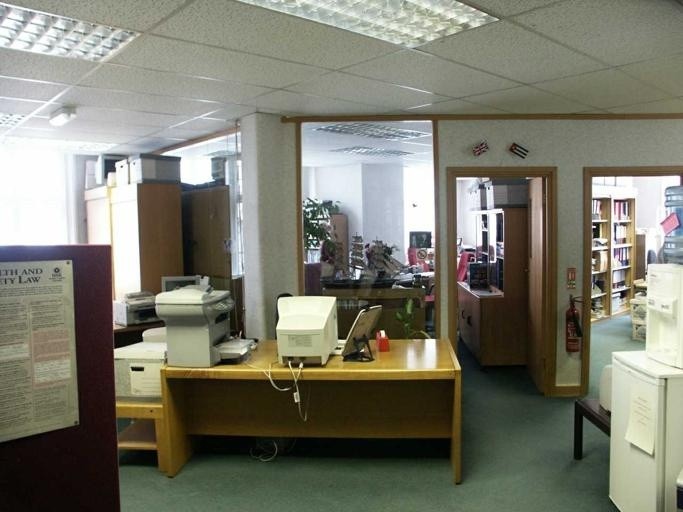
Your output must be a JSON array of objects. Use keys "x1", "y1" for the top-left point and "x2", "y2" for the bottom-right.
[
  {"x1": 113, "y1": 292, "x2": 161, "y2": 326},
  {"x1": 155, "y1": 287, "x2": 257, "y2": 368}
]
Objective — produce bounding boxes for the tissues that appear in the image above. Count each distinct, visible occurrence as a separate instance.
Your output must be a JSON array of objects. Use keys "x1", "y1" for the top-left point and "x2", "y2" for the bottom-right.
[{"x1": 376, "y1": 329, "x2": 389, "y2": 351}]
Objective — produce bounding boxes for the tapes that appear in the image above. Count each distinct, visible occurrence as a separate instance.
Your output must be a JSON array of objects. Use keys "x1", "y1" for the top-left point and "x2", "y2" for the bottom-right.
[{"x1": 379, "y1": 330, "x2": 386, "y2": 338}]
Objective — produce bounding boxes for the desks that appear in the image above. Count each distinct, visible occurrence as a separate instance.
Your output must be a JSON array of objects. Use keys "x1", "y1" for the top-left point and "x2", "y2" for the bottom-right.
[
  {"x1": 161, "y1": 339, "x2": 465, "y2": 487},
  {"x1": 321, "y1": 272, "x2": 435, "y2": 289}
]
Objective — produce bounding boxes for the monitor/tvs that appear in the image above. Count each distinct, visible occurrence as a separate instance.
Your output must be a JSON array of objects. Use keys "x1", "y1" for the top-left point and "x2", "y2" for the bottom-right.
[
  {"x1": 161, "y1": 276, "x2": 199, "y2": 292},
  {"x1": 276, "y1": 295, "x2": 339, "y2": 366}
]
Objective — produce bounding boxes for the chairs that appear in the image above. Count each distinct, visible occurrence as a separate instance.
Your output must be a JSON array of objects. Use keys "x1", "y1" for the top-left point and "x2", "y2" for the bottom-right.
[{"x1": 423, "y1": 252, "x2": 476, "y2": 333}]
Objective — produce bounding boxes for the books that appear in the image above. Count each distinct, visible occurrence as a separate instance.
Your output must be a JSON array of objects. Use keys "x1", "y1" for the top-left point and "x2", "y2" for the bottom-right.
[{"x1": 591, "y1": 200, "x2": 629, "y2": 320}]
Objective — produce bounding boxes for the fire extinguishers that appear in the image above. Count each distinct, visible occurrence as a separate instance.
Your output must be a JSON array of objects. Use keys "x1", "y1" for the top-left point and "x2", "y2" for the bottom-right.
[{"x1": 566, "y1": 294, "x2": 585, "y2": 352}]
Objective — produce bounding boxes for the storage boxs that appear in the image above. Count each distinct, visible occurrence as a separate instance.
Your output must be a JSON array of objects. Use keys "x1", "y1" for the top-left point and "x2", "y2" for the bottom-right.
[
  {"x1": 111, "y1": 342, "x2": 166, "y2": 401},
  {"x1": 465, "y1": 178, "x2": 528, "y2": 209},
  {"x1": 114, "y1": 152, "x2": 180, "y2": 185}
]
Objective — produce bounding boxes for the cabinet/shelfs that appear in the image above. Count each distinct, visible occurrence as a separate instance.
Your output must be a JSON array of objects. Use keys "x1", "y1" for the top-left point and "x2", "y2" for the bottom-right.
[
  {"x1": 590, "y1": 197, "x2": 636, "y2": 325},
  {"x1": 85, "y1": 184, "x2": 244, "y2": 333},
  {"x1": 457, "y1": 208, "x2": 528, "y2": 373}
]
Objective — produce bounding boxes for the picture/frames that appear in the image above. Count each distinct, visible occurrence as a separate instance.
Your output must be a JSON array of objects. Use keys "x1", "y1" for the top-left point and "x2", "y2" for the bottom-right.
[{"x1": 410, "y1": 231, "x2": 431, "y2": 248}]
